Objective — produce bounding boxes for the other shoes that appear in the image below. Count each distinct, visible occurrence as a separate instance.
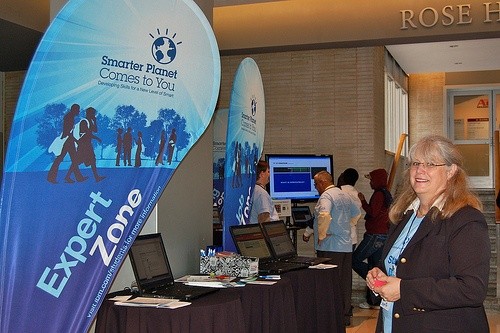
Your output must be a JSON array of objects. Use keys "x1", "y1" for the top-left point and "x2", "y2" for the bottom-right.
[{"x1": 345, "y1": 317, "x2": 350, "y2": 326}]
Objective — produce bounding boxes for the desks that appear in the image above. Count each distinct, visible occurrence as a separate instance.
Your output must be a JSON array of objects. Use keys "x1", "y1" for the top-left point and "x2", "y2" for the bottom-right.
[{"x1": 93, "y1": 260, "x2": 345, "y2": 333}]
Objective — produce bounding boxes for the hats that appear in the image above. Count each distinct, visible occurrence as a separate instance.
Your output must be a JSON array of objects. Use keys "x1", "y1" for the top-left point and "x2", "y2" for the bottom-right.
[{"x1": 364, "y1": 168, "x2": 389, "y2": 179}]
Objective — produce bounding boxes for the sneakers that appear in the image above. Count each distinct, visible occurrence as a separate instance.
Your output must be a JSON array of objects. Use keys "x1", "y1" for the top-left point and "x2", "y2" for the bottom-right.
[{"x1": 358, "y1": 301, "x2": 380, "y2": 309}]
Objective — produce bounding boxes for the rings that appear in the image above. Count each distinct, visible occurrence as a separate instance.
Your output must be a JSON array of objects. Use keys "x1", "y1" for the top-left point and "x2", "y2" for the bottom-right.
[{"x1": 380, "y1": 294, "x2": 386, "y2": 301}]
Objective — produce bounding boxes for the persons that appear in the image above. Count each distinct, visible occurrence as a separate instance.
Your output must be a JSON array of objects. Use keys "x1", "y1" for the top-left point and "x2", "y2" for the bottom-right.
[
  {"x1": 248, "y1": 161, "x2": 281, "y2": 224},
  {"x1": 365, "y1": 135, "x2": 490, "y2": 333},
  {"x1": 303, "y1": 167, "x2": 362, "y2": 326},
  {"x1": 353, "y1": 168, "x2": 393, "y2": 309}
]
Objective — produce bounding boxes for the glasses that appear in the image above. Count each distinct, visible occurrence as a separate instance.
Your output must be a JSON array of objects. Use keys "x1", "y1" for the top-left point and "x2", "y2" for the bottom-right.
[
  {"x1": 314, "y1": 181, "x2": 321, "y2": 186},
  {"x1": 406, "y1": 162, "x2": 446, "y2": 169}
]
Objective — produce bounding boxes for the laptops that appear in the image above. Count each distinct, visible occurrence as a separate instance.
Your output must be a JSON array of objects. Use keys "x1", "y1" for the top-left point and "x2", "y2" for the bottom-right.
[
  {"x1": 290, "y1": 206, "x2": 314, "y2": 227},
  {"x1": 263, "y1": 220, "x2": 334, "y2": 266},
  {"x1": 128, "y1": 232, "x2": 221, "y2": 302},
  {"x1": 229, "y1": 223, "x2": 308, "y2": 275}
]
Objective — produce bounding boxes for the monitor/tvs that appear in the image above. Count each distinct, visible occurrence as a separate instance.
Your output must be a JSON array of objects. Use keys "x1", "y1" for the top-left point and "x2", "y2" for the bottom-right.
[{"x1": 265, "y1": 154, "x2": 335, "y2": 203}]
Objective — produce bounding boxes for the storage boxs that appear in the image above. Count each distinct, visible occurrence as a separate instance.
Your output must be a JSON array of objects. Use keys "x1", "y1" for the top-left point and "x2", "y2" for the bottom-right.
[{"x1": 200, "y1": 256, "x2": 259, "y2": 278}]
